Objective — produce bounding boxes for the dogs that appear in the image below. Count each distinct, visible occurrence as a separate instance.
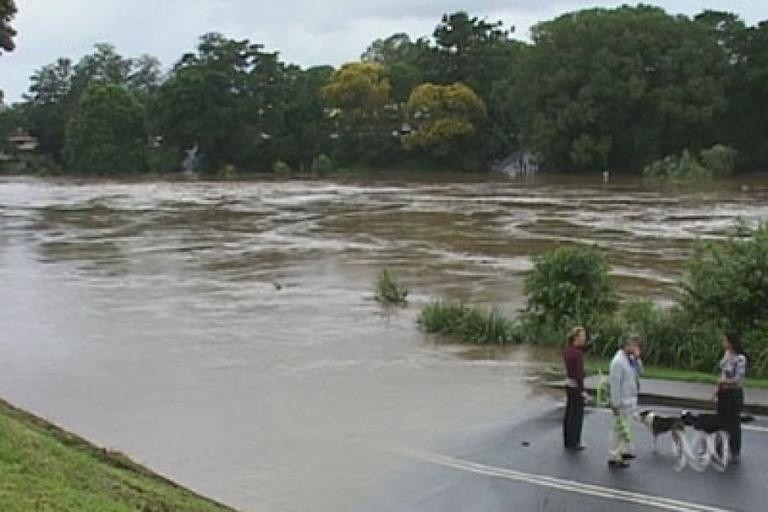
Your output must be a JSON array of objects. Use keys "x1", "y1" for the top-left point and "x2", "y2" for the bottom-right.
[
  {"x1": 639, "y1": 410, "x2": 700, "y2": 473},
  {"x1": 682, "y1": 410, "x2": 760, "y2": 472}
]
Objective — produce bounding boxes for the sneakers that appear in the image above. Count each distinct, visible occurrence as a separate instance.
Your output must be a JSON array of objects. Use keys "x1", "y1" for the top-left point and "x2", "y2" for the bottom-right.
[
  {"x1": 728, "y1": 456, "x2": 741, "y2": 464},
  {"x1": 565, "y1": 445, "x2": 586, "y2": 451},
  {"x1": 608, "y1": 452, "x2": 636, "y2": 468}
]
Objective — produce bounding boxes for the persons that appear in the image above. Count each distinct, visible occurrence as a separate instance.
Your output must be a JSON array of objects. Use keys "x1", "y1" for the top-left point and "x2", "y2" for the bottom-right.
[
  {"x1": 605, "y1": 330, "x2": 642, "y2": 469},
  {"x1": 560, "y1": 325, "x2": 590, "y2": 451},
  {"x1": 713, "y1": 328, "x2": 746, "y2": 465}
]
